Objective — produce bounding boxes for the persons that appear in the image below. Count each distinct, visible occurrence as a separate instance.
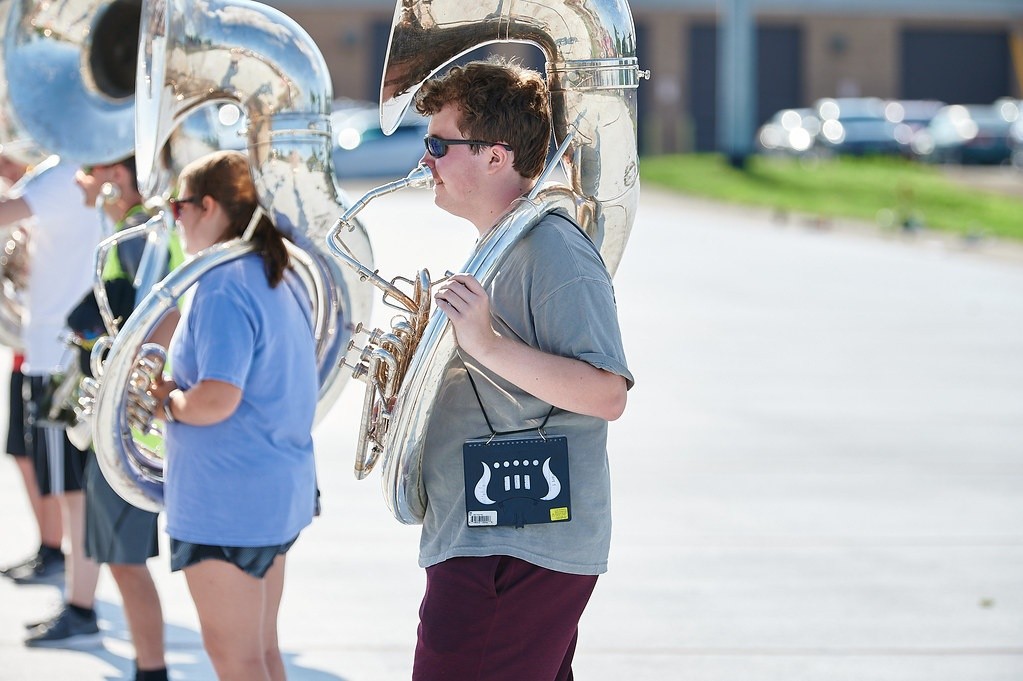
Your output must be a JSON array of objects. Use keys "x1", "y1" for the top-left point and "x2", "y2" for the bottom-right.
[
  {"x1": 0, "y1": 154, "x2": 186, "y2": 681},
  {"x1": 146, "y1": 150, "x2": 319, "y2": 681},
  {"x1": 373, "y1": 58, "x2": 636, "y2": 681}
]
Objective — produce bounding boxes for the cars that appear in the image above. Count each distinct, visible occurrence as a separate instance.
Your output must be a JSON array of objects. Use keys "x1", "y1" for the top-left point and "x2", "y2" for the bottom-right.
[
  {"x1": 326, "y1": 102, "x2": 429, "y2": 178},
  {"x1": 761, "y1": 95, "x2": 1023, "y2": 164}
]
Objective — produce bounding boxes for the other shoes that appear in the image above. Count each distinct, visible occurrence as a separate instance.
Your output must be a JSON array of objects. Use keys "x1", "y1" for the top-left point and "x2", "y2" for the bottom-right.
[
  {"x1": 25, "y1": 605, "x2": 102, "y2": 649},
  {"x1": 1, "y1": 547, "x2": 65, "y2": 582}
]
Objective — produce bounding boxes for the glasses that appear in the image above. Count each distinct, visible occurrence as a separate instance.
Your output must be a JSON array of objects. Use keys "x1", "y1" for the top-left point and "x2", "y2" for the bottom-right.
[
  {"x1": 168, "y1": 192, "x2": 219, "y2": 220},
  {"x1": 424, "y1": 134, "x2": 514, "y2": 158},
  {"x1": 81, "y1": 163, "x2": 115, "y2": 177}
]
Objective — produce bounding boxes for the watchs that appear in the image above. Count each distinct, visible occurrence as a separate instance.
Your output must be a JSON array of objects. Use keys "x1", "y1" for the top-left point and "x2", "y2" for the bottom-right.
[{"x1": 162, "y1": 388, "x2": 184, "y2": 424}]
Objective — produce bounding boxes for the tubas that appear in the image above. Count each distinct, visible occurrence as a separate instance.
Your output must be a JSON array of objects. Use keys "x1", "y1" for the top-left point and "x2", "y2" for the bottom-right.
[{"x1": 1, "y1": 0, "x2": 177, "y2": 456}]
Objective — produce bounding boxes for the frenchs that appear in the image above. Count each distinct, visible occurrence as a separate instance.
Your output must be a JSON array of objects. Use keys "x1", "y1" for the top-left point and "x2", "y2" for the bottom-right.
[
  {"x1": 322, "y1": 1, "x2": 649, "y2": 530},
  {"x1": 75, "y1": 0, "x2": 385, "y2": 517},
  {"x1": 0, "y1": 0, "x2": 142, "y2": 172}
]
